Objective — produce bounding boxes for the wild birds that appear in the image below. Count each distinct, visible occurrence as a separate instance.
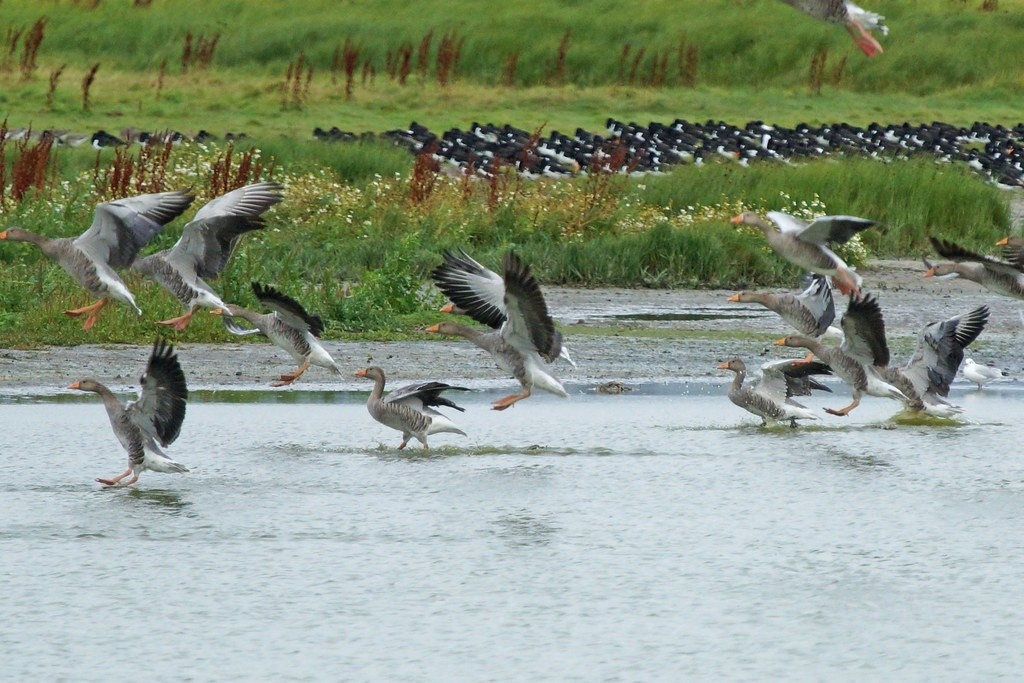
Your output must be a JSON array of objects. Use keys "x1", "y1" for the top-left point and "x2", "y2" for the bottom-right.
[
  {"x1": 0, "y1": 115, "x2": 1024, "y2": 193},
  {"x1": 777, "y1": 1, "x2": 891, "y2": 60},
  {"x1": 0, "y1": 175, "x2": 1024, "y2": 494}
]
